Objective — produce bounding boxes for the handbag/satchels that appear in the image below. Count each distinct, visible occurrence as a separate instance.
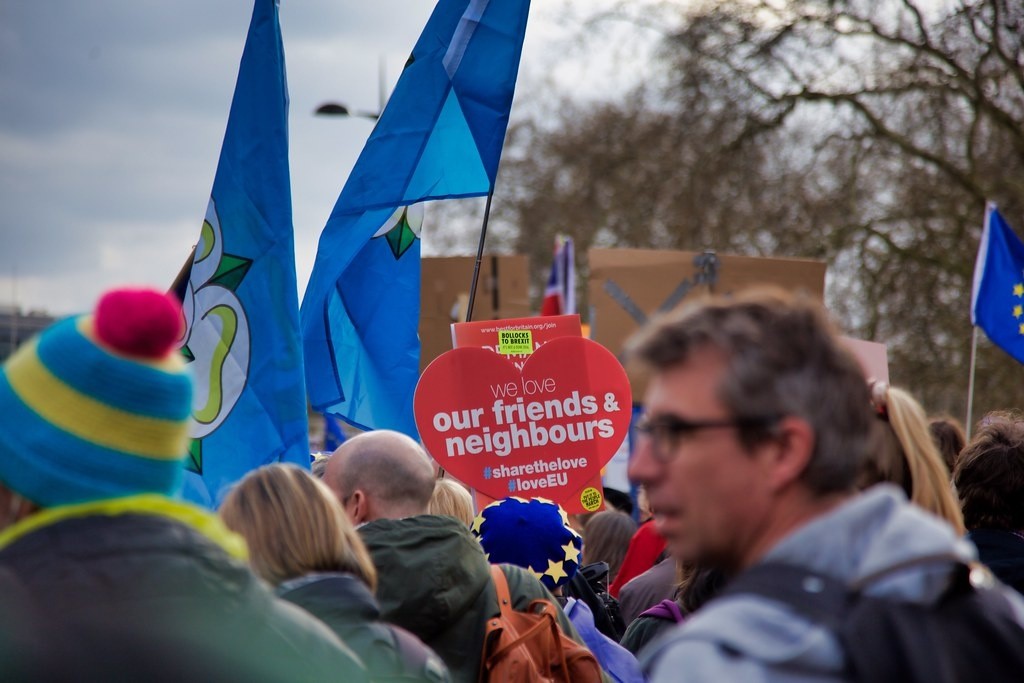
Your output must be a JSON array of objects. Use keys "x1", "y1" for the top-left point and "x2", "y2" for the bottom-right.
[
  {"x1": 596, "y1": 587, "x2": 627, "y2": 640},
  {"x1": 479, "y1": 564, "x2": 604, "y2": 683}
]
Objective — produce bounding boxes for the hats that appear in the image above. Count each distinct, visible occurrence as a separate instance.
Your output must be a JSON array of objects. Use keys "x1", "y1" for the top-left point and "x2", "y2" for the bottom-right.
[
  {"x1": 470, "y1": 495, "x2": 583, "y2": 591},
  {"x1": 0, "y1": 288, "x2": 196, "y2": 509}
]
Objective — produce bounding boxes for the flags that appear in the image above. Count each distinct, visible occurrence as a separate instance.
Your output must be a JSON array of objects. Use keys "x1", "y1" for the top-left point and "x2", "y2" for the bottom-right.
[
  {"x1": 301, "y1": 0, "x2": 532, "y2": 444},
  {"x1": 542, "y1": 239, "x2": 576, "y2": 316},
  {"x1": 163, "y1": 0, "x2": 312, "y2": 512},
  {"x1": 972, "y1": 207, "x2": 1024, "y2": 363}
]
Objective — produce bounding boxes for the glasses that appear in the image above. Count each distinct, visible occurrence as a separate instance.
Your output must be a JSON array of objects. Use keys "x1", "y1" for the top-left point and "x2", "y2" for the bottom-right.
[{"x1": 625, "y1": 410, "x2": 789, "y2": 462}]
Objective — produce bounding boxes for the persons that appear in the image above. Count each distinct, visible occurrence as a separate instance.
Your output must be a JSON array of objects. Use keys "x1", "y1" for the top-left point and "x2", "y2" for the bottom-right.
[
  {"x1": 626, "y1": 289, "x2": 1023, "y2": 683},
  {"x1": 220, "y1": 429, "x2": 692, "y2": 683},
  {"x1": 0, "y1": 287, "x2": 367, "y2": 683}
]
